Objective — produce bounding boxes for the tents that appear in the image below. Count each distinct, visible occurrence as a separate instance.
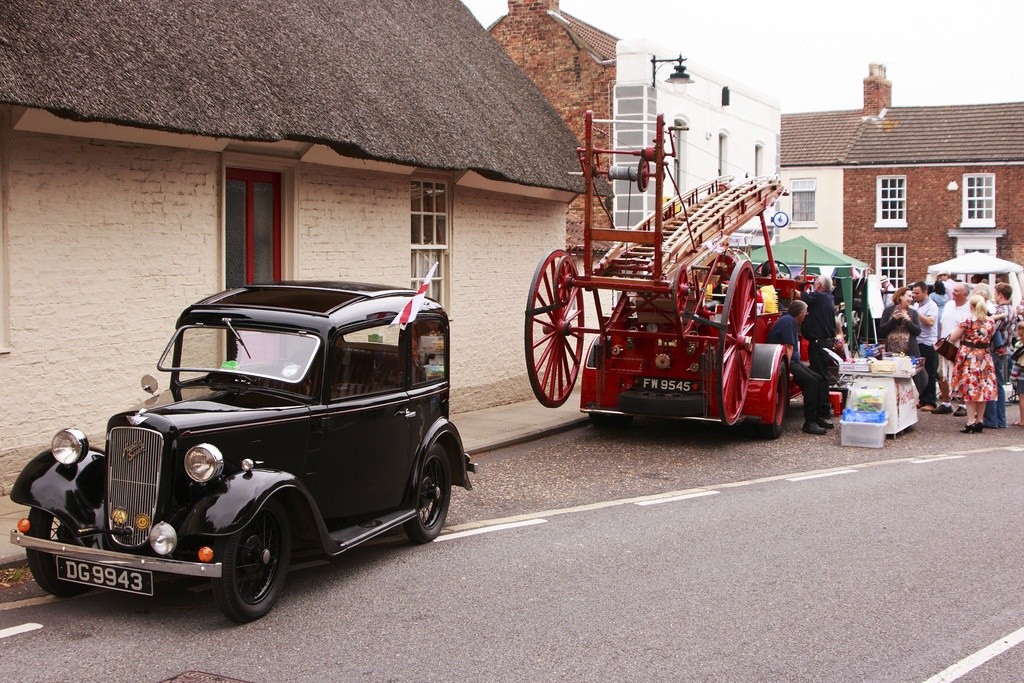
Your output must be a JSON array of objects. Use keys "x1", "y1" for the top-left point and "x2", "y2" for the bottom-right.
[
  {"x1": 925, "y1": 250, "x2": 1024, "y2": 310},
  {"x1": 737, "y1": 236, "x2": 871, "y2": 358}
]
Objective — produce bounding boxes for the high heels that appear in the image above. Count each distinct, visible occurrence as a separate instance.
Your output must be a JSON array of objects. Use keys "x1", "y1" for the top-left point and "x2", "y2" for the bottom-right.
[
  {"x1": 976, "y1": 422, "x2": 983, "y2": 433},
  {"x1": 960, "y1": 423, "x2": 976, "y2": 433}
]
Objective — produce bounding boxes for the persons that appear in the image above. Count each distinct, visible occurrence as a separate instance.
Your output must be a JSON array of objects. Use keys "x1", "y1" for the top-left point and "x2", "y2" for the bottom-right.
[
  {"x1": 767, "y1": 300, "x2": 835, "y2": 436},
  {"x1": 767, "y1": 273, "x2": 837, "y2": 420},
  {"x1": 878, "y1": 272, "x2": 1024, "y2": 435}
]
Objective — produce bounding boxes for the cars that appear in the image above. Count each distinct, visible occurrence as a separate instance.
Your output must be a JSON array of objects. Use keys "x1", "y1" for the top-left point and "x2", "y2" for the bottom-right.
[{"x1": 9, "y1": 282, "x2": 479, "y2": 623}]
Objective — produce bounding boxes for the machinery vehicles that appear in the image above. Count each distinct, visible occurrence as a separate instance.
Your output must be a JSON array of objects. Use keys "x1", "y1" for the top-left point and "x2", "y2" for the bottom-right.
[{"x1": 527, "y1": 110, "x2": 843, "y2": 440}]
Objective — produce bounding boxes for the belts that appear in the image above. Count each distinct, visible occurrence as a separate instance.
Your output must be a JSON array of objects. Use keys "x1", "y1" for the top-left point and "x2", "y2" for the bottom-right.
[{"x1": 816, "y1": 338, "x2": 831, "y2": 341}]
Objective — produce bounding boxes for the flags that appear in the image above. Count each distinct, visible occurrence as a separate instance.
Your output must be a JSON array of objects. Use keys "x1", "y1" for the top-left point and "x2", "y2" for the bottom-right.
[{"x1": 388, "y1": 261, "x2": 439, "y2": 331}]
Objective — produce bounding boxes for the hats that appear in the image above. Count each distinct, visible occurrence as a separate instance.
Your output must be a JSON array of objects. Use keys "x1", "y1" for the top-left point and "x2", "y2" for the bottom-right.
[
  {"x1": 937, "y1": 269, "x2": 951, "y2": 275},
  {"x1": 880, "y1": 275, "x2": 888, "y2": 283}
]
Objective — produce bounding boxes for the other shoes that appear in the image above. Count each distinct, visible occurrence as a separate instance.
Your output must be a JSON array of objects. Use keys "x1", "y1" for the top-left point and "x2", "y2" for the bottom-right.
[
  {"x1": 921, "y1": 404, "x2": 935, "y2": 411},
  {"x1": 931, "y1": 404, "x2": 953, "y2": 414},
  {"x1": 816, "y1": 419, "x2": 834, "y2": 429},
  {"x1": 916, "y1": 404, "x2": 925, "y2": 409},
  {"x1": 802, "y1": 422, "x2": 826, "y2": 434},
  {"x1": 954, "y1": 407, "x2": 967, "y2": 416}
]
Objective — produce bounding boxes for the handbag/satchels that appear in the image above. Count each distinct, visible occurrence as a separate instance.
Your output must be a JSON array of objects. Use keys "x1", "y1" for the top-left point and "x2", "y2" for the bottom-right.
[{"x1": 934, "y1": 333, "x2": 958, "y2": 363}]
